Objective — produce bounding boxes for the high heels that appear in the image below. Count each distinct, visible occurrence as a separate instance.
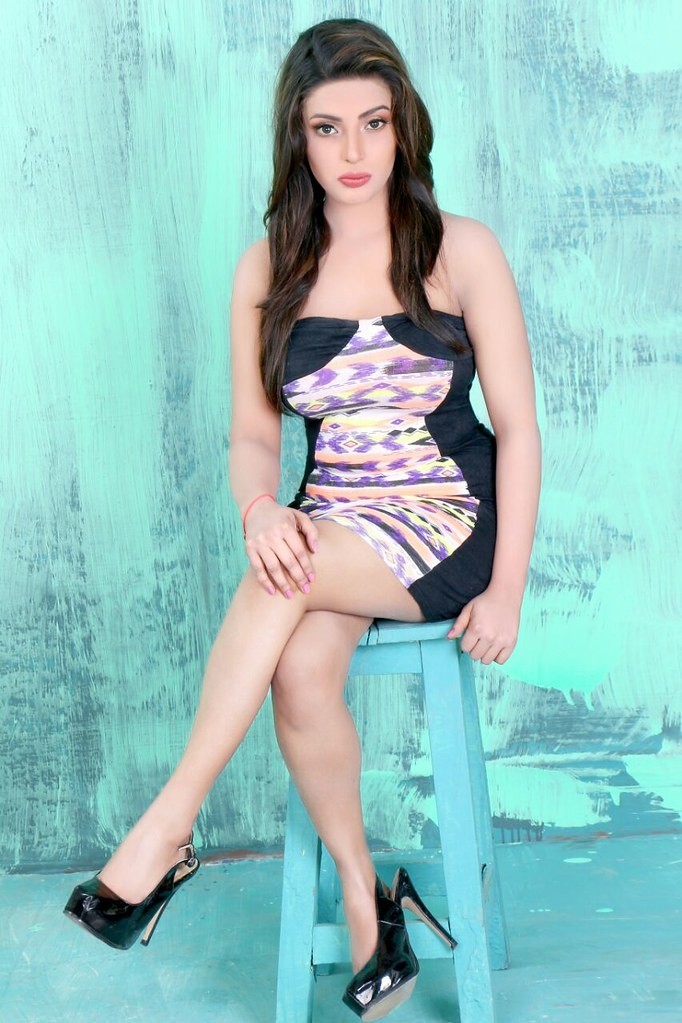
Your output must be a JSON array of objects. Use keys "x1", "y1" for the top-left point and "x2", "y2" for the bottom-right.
[
  {"x1": 63, "y1": 829, "x2": 200, "y2": 949},
  {"x1": 342, "y1": 865, "x2": 458, "y2": 1021}
]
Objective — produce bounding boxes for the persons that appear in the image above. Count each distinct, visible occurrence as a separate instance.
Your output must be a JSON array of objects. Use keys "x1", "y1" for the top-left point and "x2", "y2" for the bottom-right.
[{"x1": 65, "y1": 16, "x2": 541, "y2": 1022}]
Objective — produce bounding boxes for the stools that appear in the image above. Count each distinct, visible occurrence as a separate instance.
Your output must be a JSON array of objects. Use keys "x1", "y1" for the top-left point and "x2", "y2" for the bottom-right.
[{"x1": 273, "y1": 618, "x2": 509, "y2": 1023}]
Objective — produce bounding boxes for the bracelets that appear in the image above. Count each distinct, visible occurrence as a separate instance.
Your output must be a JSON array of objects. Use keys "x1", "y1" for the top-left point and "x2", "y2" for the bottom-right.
[{"x1": 244, "y1": 494, "x2": 274, "y2": 539}]
